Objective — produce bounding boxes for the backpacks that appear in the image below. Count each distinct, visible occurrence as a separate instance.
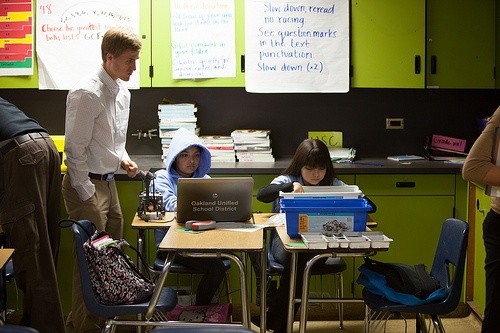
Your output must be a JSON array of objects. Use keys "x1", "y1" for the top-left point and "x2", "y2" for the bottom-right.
[{"x1": 365, "y1": 255, "x2": 442, "y2": 301}]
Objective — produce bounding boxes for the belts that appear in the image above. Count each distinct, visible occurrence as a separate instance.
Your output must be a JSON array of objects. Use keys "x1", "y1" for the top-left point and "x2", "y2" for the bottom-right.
[
  {"x1": 0, "y1": 131, "x2": 50, "y2": 157},
  {"x1": 88, "y1": 172, "x2": 116, "y2": 182}
]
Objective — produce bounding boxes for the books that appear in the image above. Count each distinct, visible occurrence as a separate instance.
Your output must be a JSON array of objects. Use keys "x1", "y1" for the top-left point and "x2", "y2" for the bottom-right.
[
  {"x1": 158, "y1": 104, "x2": 200, "y2": 162},
  {"x1": 198, "y1": 135, "x2": 237, "y2": 162},
  {"x1": 231, "y1": 130, "x2": 274, "y2": 163},
  {"x1": 387, "y1": 155, "x2": 424, "y2": 161},
  {"x1": 427, "y1": 145, "x2": 468, "y2": 160}
]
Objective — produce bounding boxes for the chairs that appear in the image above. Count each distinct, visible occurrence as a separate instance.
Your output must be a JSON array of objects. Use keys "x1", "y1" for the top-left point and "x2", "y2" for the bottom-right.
[
  {"x1": 268, "y1": 227, "x2": 345, "y2": 332},
  {"x1": 152, "y1": 228, "x2": 234, "y2": 323},
  {"x1": 363, "y1": 220, "x2": 468, "y2": 333},
  {"x1": 73, "y1": 218, "x2": 177, "y2": 333}
]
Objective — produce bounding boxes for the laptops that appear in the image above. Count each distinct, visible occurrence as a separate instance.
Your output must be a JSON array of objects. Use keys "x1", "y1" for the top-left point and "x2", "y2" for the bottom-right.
[{"x1": 174, "y1": 178, "x2": 254, "y2": 222}]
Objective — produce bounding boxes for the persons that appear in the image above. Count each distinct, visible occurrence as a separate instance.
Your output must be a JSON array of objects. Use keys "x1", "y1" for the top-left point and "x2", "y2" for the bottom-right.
[
  {"x1": 462, "y1": 106, "x2": 500, "y2": 333},
  {"x1": 63, "y1": 28, "x2": 142, "y2": 333},
  {"x1": 0, "y1": 97, "x2": 65, "y2": 333},
  {"x1": 257, "y1": 139, "x2": 377, "y2": 333},
  {"x1": 149, "y1": 127, "x2": 232, "y2": 306}
]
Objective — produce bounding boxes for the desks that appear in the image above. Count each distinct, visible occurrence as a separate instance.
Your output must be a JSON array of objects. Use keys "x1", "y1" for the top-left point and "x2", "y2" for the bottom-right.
[
  {"x1": 274, "y1": 225, "x2": 389, "y2": 333},
  {"x1": 132, "y1": 209, "x2": 255, "y2": 333},
  {"x1": 145, "y1": 225, "x2": 265, "y2": 333},
  {"x1": 251, "y1": 212, "x2": 377, "y2": 330}
]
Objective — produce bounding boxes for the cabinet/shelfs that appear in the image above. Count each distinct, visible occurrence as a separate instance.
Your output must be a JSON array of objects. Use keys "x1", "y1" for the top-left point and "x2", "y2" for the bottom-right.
[
  {"x1": 0, "y1": 0, "x2": 500, "y2": 89},
  {"x1": 5, "y1": 172, "x2": 492, "y2": 322}
]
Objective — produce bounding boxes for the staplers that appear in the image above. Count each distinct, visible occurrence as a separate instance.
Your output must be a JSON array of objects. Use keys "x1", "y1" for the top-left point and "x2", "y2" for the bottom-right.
[{"x1": 185, "y1": 220, "x2": 216, "y2": 231}]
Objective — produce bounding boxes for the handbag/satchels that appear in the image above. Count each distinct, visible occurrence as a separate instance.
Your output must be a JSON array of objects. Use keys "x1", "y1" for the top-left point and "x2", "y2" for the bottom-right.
[
  {"x1": 57, "y1": 217, "x2": 157, "y2": 305},
  {"x1": 167, "y1": 302, "x2": 232, "y2": 324}
]
